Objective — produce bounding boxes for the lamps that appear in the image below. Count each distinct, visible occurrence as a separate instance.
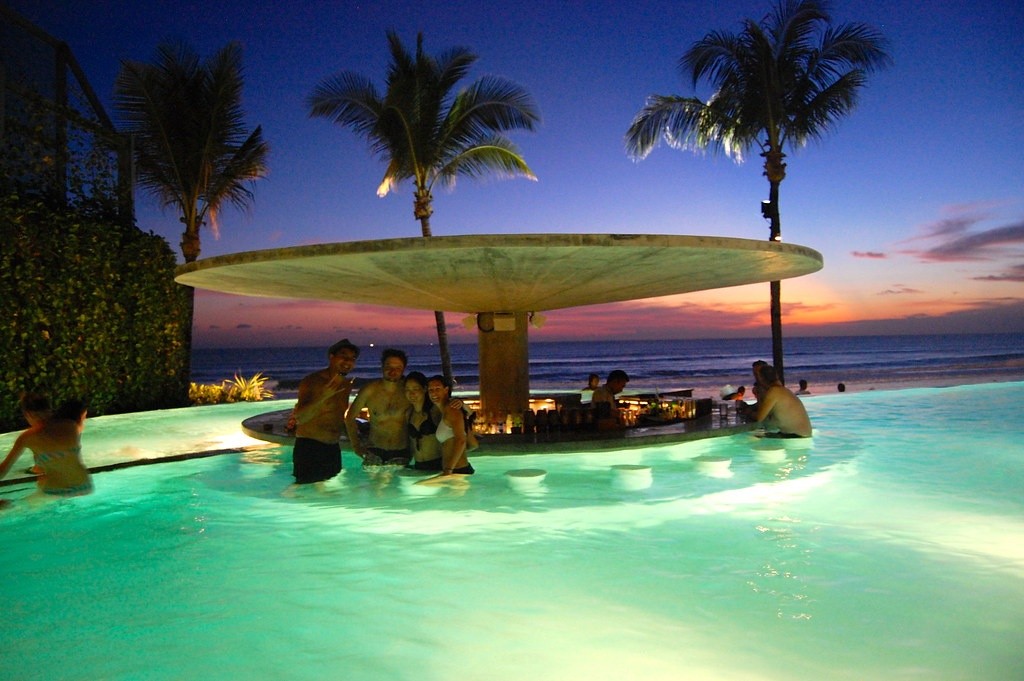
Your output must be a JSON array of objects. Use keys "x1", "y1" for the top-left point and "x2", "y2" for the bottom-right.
[
  {"x1": 462, "y1": 313, "x2": 478, "y2": 329},
  {"x1": 529, "y1": 312, "x2": 545, "y2": 329}
]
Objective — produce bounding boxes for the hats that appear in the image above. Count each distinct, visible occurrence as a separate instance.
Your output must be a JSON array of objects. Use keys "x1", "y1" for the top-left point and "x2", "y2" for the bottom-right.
[{"x1": 328, "y1": 339, "x2": 359, "y2": 360}]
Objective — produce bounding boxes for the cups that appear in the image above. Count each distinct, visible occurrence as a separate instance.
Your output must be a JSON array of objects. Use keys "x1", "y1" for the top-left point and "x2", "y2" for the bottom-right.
[
  {"x1": 727, "y1": 400, "x2": 737, "y2": 419},
  {"x1": 712, "y1": 399, "x2": 720, "y2": 415},
  {"x1": 719, "y1": 400, "x2": 728, "y2": 415}
]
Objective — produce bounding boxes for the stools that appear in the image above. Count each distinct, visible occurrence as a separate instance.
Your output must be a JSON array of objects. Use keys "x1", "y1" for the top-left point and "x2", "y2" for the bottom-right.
[
  {"x1": 695, "y1": 457, "x2": 732, "y2": 471},
  {"x1": 781, "y1": 438, "x2": 813, "y2": 451},
  {"x1": 751, "y1": 445, "x2": 786, "y2": 464},
  {"x1": 507, "y1": 469, "x2": 546, "y2": 489},
  {"x1": 609, "y1": 465, "x2": 653, "y2": 492},
  {"x1": 401, "y1": 468, "x2": 444, "y2": 497}
]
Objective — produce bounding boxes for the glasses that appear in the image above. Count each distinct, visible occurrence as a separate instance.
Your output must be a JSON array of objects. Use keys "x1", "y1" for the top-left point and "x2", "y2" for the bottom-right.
[{"x1": 336, "y1": 353, "x2": 356, "y2": 362}]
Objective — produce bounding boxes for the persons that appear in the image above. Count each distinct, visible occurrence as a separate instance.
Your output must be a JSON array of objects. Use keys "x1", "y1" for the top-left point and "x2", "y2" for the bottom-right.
[
  {"x1": 592, "y1": 370, "x2": 630, "y2": 431},
  {"x1": 838, "y1": 383, "x2": 845, "y2": 392},
  {"x1": 737, "y1": 364, "x2": 812, "y2": 439},
  {"x1": 752, "y1": 359, "x2": 777, "y2": 430},
  {"x1": 404, "y1": 371, "x2": 464, "y2": 468},
  {"x1": 0, "y1": 391, "x2": 93, "y2": 504},
  {"x1": 341, "y1": 349, "x2": 464, "y2": 466},
  {"x1": 427, "y1": 375, "x2": 475, "y2": 476},
  {"x1": 793, "y1": 379, "x2": 811, "y2": 396},
  {"x1": 25, "y1": 397, "x2": 88, "y2": 476},
  {"x1": 291, "y1": 338, "x2": 360, "y2": 492},
  {"x1": 582, "y1": 374, "x2": 602, "y2": 391}
]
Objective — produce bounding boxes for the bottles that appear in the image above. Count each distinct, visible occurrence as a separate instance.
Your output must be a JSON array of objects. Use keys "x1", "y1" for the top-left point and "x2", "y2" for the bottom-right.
[
  {"x1": 617, "y1": 396, "x2": 697, "y2": 426},
  {"x1": 470, "y1": 409, "x2": 523, "y2": 436}
]
